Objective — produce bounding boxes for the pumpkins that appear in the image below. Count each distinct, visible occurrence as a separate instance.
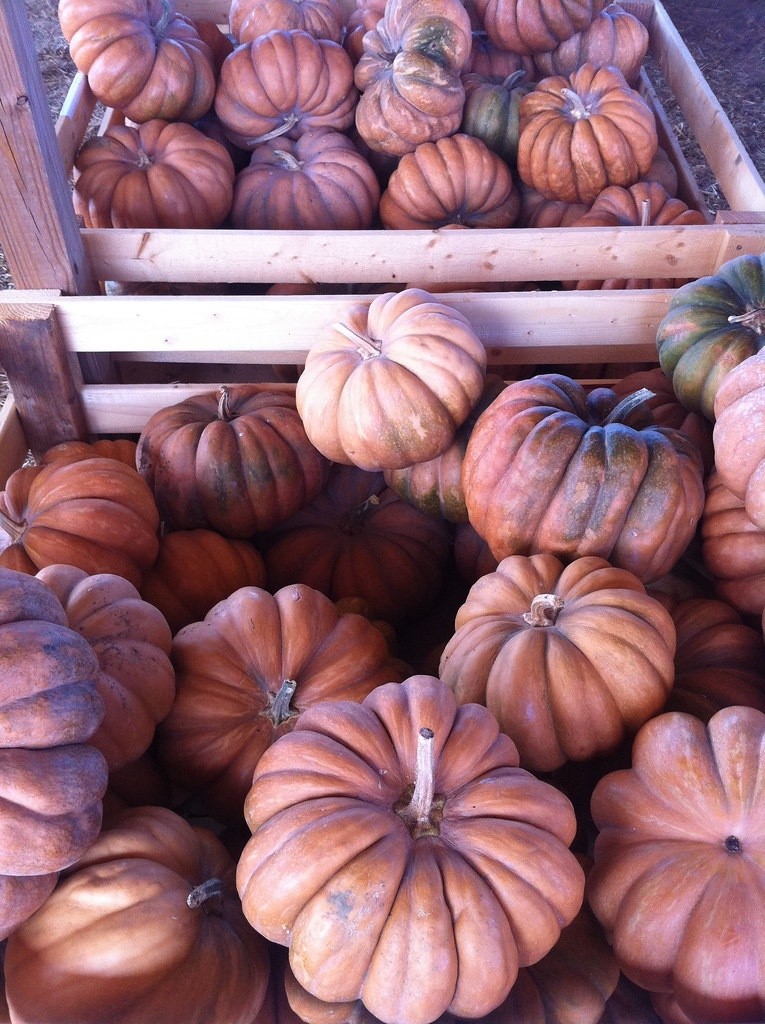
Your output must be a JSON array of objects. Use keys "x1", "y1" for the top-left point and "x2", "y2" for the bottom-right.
[{"x1": 0, "y1": 0, "x2": 765, "y2": 1024}]
